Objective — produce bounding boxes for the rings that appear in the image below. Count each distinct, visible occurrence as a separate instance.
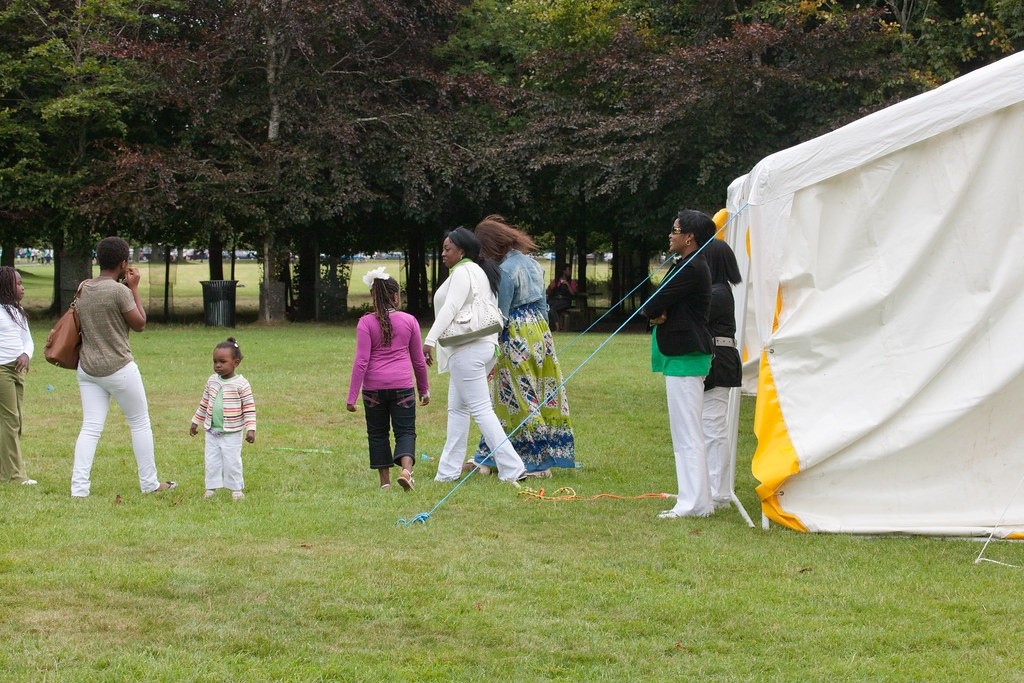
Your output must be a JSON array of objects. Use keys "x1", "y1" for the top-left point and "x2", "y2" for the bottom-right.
[{"x1": 426, "y1": 352, "x2": 430, "y2": 359}]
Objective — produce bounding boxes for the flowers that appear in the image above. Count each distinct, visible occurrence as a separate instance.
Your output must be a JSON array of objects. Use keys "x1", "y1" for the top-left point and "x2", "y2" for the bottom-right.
[{"x1": 362, "y1": 266, "x2": 391, "y2": 290}]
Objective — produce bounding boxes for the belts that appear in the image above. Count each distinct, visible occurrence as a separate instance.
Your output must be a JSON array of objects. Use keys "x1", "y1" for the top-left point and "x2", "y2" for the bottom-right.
[{"x1": 713, "y1": 336, "x2": 737, "y2": 347}]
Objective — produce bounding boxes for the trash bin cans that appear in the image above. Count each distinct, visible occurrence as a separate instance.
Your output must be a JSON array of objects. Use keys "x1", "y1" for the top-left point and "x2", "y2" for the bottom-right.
[{"x1": 200, "y1": 280, "x2": 238, "y2": 328}]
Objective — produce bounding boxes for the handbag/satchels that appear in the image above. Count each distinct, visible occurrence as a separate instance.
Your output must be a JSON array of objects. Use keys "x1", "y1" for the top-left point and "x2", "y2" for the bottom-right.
[
  {"x1": 45, "y1": 280, "x2": 87, "y2": 369},
  {"x1": 437, "y1": 267, "x2": 506, "y2": 347}
]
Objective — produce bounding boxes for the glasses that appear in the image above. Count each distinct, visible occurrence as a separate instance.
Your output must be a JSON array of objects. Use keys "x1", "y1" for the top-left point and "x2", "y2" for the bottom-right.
[{"x1": 671, "y1": 225, "x2": 690, "y2": 236}]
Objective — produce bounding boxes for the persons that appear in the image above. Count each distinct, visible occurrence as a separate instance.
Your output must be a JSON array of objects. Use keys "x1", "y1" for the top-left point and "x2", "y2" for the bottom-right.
[
  {"x1": 347, "y1": 266, "x2": 430, "y2": 493},
  {"x1": 190, "y1": 338, "x2": 256, "y2": 500},
  {"x1": 546, "y1": 266, "x2": 577, "y2": 332},
  {"x1": 645, "y1": 209, "x2": 742, "y2": 519},
  {"x1": 467, "y1": 214, "x2": 575, "y2": 479},
  {"x1": 423, "y1": 226, "x2": 527, "y2": 490},
  {"x1": 0, "y1": 266, "x2": 37, "y2": 486},
  {"x1": 71, "y1": 237, "x2": 178, "y2": 497}
]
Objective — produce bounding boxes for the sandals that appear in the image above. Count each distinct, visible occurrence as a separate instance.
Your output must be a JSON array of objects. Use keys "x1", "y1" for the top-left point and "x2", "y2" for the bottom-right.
[
  {"x1": 381, "y1": 485, "x2": 389, "y2": 491},
  {"x1": 398, "y1": 473, "x2": 416, "y2": 492}
]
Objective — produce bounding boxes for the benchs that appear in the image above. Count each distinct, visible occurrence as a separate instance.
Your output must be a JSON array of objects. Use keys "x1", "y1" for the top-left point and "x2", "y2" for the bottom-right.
[{"x1": 560, "y1": 306, "x2": 615, "y2": 332}]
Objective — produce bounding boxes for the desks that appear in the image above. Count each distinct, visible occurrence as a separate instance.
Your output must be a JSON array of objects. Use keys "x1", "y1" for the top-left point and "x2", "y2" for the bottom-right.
[{"x1": 572, "y1": 292, "x2": 604, "y2": 329}]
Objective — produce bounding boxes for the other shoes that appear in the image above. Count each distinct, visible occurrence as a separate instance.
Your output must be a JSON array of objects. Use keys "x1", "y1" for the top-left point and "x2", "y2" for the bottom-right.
[
  {"x1": 232, "y1": 490, "x2": 245, "y2": 500},
  {"x1": 205, "y1": 490, "x2": 213, "y2": 500},
  {"x1": 21, "y1": 479, "x2": 37, "y2": 485},
  {"x1": 157, "y1": 481, "x2": 177, "y2": 492},
  {"x1": 659, "y1": 504, "x2": 714, "y2": 519},
  {"x1": 467, "y1": 458, "x2": 491, "y2": 476}
]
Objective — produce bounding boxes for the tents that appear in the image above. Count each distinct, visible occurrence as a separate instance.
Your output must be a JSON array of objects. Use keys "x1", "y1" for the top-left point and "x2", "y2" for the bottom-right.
[{"x1": 711, "y1": 50, "x2": 1023, "y2": 539}]
